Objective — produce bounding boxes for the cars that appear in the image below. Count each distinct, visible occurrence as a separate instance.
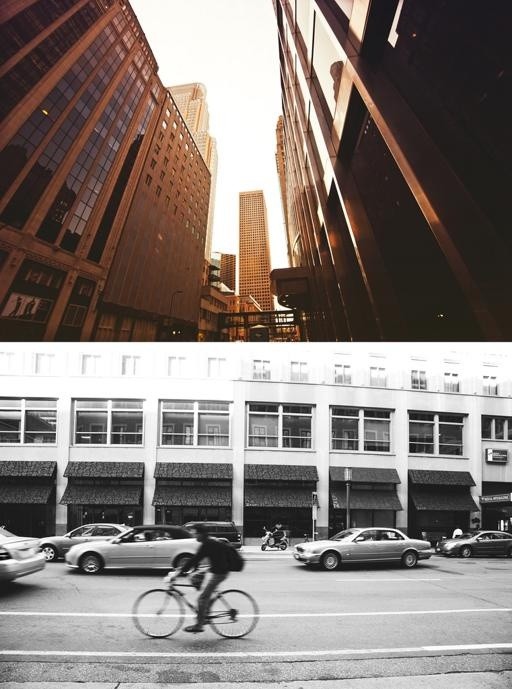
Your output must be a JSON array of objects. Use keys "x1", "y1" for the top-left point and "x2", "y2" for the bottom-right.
[
  {"x1": 0, "y1": 520, "x2": 214, "y2": 584},
  {"x1": 435, "y1": 530, "x2": 512, "y2": 560},
  {"x1": 291, "y1": 528, "x2": 433, "y2": 571}
]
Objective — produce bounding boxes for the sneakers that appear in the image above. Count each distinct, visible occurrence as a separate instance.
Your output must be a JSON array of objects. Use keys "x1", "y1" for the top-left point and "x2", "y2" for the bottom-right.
[{"x1": 185, "y1": 625, "x2": 203, "y2": 632}]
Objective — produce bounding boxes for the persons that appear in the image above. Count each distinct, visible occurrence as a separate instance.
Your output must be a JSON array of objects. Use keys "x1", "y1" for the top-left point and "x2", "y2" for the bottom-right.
[
  {"x1": 162, "y1": 523, "x2": 232, "y2": 633},
  {"x1": 269, "y1": 522, "x2": 287, "y2": 545}
]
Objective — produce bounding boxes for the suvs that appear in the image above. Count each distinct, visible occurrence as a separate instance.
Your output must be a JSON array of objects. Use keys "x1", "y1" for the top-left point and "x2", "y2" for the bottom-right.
[{"x1": 181, "y1": 519, "x2": 242, "y2": 552}]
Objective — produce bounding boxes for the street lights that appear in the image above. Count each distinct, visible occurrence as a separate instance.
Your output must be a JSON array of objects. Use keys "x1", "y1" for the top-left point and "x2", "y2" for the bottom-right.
[{"x1": 343, "y1": 464, "x2": 355, "y2": 530}]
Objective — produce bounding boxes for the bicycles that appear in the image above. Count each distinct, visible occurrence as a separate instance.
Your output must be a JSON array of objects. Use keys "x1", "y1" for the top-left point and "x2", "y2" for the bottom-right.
[{"x1": 132, "y1": 563, "x2": 260, "y2": 643}]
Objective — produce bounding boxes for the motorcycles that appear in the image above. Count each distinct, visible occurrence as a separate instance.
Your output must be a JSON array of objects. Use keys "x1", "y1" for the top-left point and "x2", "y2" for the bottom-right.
[{"x1": 259, "y1": 526, "x2": 289, "y2": 552}]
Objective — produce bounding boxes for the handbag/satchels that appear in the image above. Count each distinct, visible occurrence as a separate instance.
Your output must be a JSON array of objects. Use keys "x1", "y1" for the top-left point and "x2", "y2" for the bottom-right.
[{"x1": 226, "y1": 544, "x2": 243, "y2": 571}]
